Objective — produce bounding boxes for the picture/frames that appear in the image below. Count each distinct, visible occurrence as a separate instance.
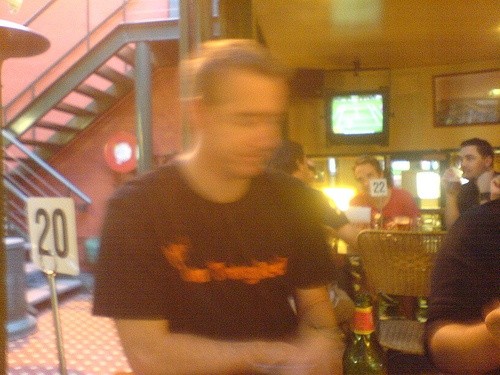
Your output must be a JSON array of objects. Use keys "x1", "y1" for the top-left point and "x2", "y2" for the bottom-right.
[{"x1": 432, "y1": 69, "x2": 500, "y2": 128}]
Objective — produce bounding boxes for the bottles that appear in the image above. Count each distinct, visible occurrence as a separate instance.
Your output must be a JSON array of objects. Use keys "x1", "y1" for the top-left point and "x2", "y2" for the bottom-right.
[{"x1": 343, "y1": 295, "x2": 388, "y2": 375}]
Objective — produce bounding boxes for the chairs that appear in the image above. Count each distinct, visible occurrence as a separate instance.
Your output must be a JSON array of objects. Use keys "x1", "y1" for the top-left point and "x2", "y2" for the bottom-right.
[{"x1": 317, "y1": 209, "x2": 447, "y2": 375}]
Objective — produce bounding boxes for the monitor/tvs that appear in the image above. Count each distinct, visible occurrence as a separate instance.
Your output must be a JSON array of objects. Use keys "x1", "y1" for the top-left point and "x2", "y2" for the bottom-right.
[{"x1": 324, "y1": 90, "x2": 389, "y2": 147}]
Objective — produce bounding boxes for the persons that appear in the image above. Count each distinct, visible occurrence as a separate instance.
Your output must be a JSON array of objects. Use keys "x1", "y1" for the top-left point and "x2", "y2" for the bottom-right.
[
  {"x1": 350, "y1": 153, "x2": 422, "y2": 230},
  {"x1": 271, "y1": 140, "x2": 360, "y2": 324},
  {"x1": 422, "y1": 199, "x2": 500, "y2": 375},
  {"x1": 441, "y1": 138, "x2": 500, "y2": 233},
  {"x1": 89, "y1": 38, "x2": 345, "y2": 375},
  {"x1": 304, "y1": 162, "x2": 316, "y2": 190}
]
